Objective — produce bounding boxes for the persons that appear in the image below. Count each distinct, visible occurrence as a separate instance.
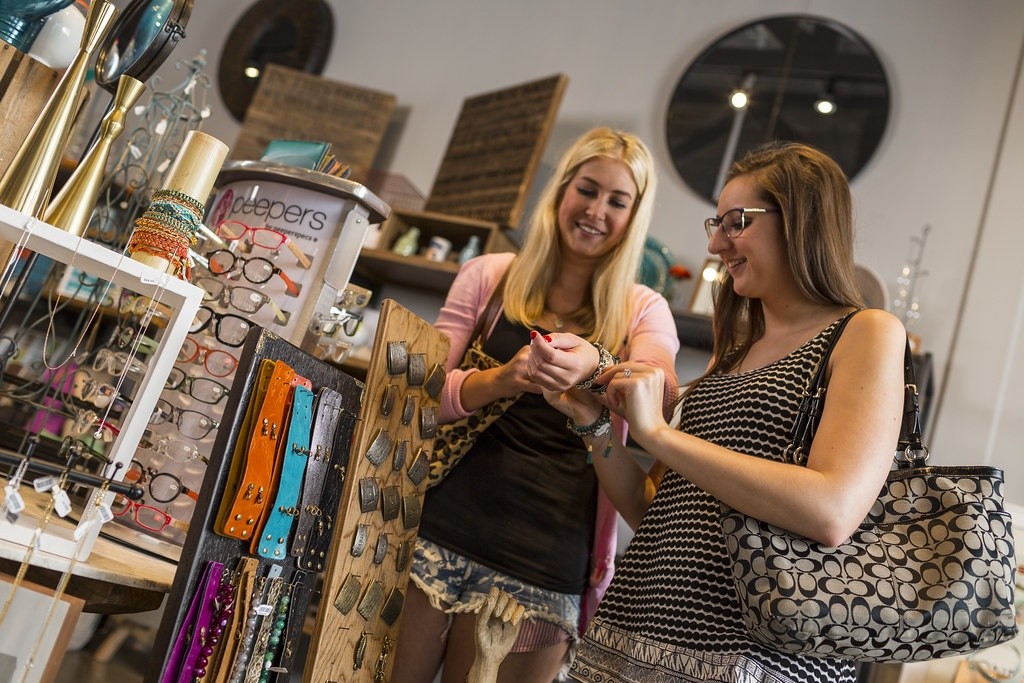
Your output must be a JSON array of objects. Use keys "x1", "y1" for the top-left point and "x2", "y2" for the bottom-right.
[
  {"x1": 390, "y1": 127, "x2": 680, "y2": 683},
  {"x1": 468, "y1": 586, "x2": 526, "y2": 683},
  {"x1": 567, "y1": 139, "x2": 908, "y2": 683}
]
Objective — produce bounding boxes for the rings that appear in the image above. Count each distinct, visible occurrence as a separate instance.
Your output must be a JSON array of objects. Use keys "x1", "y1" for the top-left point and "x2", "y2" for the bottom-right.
[{"x1": 623, "y1": 367, "x2": 631, "y2": 377}]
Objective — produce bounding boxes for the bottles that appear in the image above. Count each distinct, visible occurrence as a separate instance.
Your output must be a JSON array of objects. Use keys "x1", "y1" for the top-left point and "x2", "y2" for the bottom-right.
[
  {"x1": 391, "y1": 226, "x2": 421, "y2": 256},
  {"x1": 457, "y1": 236, "x2": 479, "y2": 264}
]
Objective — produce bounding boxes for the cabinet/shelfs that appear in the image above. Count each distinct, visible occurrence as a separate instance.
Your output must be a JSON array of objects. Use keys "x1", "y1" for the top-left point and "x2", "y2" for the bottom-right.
[{"x1": 309, "y1": 205, "x2": 522, "y2": 376}]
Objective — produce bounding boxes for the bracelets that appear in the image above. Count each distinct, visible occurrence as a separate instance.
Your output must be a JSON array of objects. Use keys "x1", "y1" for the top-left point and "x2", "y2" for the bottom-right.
[
  {"x1": 577, "y1": 342, "x2": 611, "y2": 394},
  {"x1": 127, "y1": 190, "x2": 203, "y2": 265},
  {"x1": 566, "y1": 405, "x2": 615, "y2": 463},
  {"x1": 590, "y1": 352, "x2": 624, "y2": 392}
]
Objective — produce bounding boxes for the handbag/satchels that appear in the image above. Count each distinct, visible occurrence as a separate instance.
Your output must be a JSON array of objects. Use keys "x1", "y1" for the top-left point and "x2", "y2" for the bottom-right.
[
  {"x1": 425, "y1": 252, "x2": 527, "y2": 491},
  {"x1": 717, "y1": 308, "x2": 1019, "y2": 665}
]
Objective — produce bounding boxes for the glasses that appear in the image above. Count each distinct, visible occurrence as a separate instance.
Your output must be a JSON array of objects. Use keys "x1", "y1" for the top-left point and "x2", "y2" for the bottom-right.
[
  {"x1": 216, "y1": 219, "x2": 311, "y2": 270},
  {"x1": 93, "y1": 349, "x2": 148, "y2": 376},
  {"x1": 313, "y1": 283, "x2": 373, "y2": 363},
  {"x1": 107, "y1": 326, "x2": 156, "y2": 354},
  {"x1": 704, "y1": 207, "x2": 785, "y2": 241},
  {"x1": 163, "y1": 368, "x2": 232, "y2": 404},
  {"x1": 81, "y1": 379, "x2": 131, "y2": 408},
  {"x1": 137, "y1": 428, "x2": 210, "y2": 466},
  {"x1": 205, "y1": 248, "x2": 299, "y2": 295},
  {"x1": 193, "y1": 274, "x2": 287, "y2": 326},
  {"x1": 148, "y1": 399, "x2": 220, "y2": 440},
  {"x1": 175, "y1": 337, "x2": 240, "y2": 378},
  {"x1": 187, "y1": 305, "x2": 259, "y2": 348},
  {"x1": 71, "y1": 408, "x2": 120, "y2": 436},
  {"x1": 110, "y1": 494, "x2": 191, "y2": 534},
  {"x1": 119, "y1": 294, "x2": 170, "y2": 323},
  {"x1": 124, "y1": 459, "x2": 198, "y2": 504},
  {"x1": 57, "y1": 435, "x2": 113, "y2": 465}
]
